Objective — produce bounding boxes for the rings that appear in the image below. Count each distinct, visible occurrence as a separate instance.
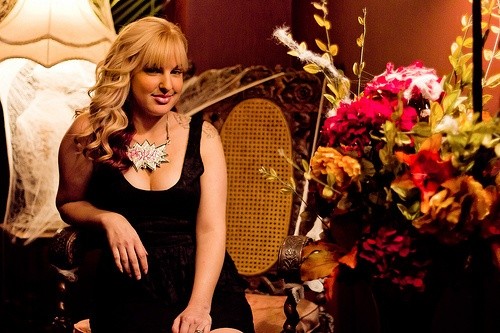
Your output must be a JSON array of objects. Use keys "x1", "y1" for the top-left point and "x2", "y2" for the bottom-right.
[{"x1": 195, "y1": 330, "x2": 202, "y2": 333}]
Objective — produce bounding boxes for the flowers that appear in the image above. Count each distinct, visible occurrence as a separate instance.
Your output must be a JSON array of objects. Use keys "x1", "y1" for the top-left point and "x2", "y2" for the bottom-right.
[{"x1": 260, "y1": 0, "x2": 500, "y2": 294}]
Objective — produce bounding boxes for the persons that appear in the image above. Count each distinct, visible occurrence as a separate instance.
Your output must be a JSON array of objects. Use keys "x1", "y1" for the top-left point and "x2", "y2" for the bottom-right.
[{"x1": 55, "y1": 16, "x2": 255, "y2": 333}]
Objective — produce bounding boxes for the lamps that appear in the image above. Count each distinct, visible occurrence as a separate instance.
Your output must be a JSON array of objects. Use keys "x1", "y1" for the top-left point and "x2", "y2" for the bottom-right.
[{"x1": 0, "y1": 0, "x2": 120, "y2": 242}]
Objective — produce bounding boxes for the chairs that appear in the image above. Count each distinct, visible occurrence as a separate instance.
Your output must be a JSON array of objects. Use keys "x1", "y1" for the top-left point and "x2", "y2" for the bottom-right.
[{"x1": 52, "y1": 64, "x2": 335, "y2": 333}]
